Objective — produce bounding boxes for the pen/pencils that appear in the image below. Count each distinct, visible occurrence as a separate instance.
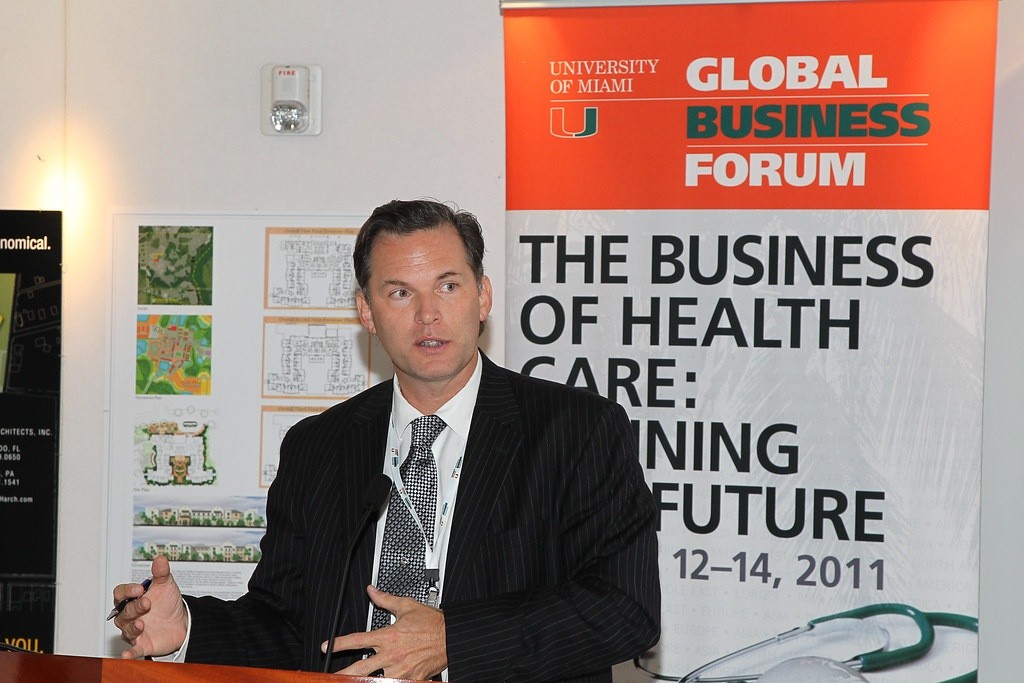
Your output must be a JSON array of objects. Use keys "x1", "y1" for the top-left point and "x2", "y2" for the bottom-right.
[{"x1": 108, "y1": 575, "x2": 153, "y2": 623}]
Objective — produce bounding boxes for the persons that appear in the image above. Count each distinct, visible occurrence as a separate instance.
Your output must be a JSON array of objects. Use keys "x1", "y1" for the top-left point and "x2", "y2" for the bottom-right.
[{"x1": 113, "y1": 197, "x2": 662, "y2": 683}]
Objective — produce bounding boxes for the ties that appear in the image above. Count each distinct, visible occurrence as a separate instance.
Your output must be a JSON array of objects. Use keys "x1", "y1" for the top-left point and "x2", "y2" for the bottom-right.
[{"x1": 365, "y1": 415, "x2": 449, "y2": 681}]
